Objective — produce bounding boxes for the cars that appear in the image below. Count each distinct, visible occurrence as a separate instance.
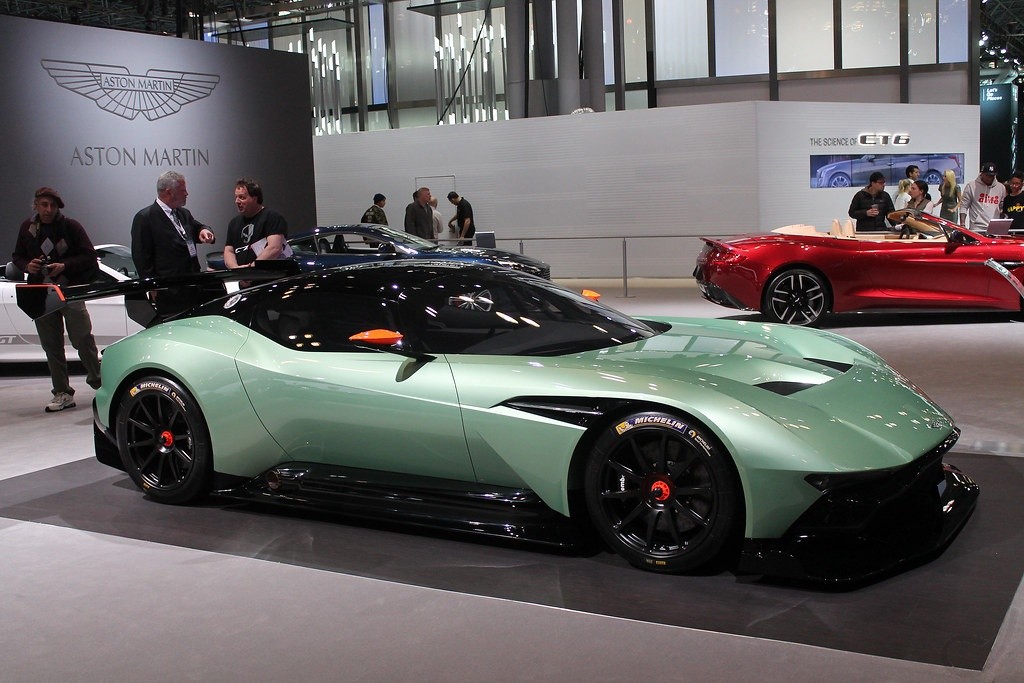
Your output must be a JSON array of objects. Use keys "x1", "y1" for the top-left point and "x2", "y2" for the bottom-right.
[{"x1": 817, "y1": 153, "x2": 963, "y2": 187}]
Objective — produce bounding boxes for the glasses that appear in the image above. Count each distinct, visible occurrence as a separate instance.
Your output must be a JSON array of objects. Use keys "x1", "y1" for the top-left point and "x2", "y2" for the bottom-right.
[{"x1": 876, "y1": 181, "x2": 885, "y2": 185}]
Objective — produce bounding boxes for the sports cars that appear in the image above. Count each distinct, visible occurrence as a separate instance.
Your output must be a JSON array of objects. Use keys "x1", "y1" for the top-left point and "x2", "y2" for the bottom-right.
[
  {"x1": 693, "y1": 208, "x2": 1024, "y2": 329},
  {"x1": 92, "y1": 258, "x2": 981, "y2": 592},
  {"x1": 204, "y1": 223, "x2": 551, "y2": 284},
  {"x1": 0, "y1": 244, "x2": 250, "y2": 363}
]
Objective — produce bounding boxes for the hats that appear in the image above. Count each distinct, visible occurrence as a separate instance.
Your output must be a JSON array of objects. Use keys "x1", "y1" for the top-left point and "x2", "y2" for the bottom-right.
[
  {"x1": 35, "y1": 187, "x2": 64, "y2": 209},
  {"x1": 870, "y1": 172, "x2": 884, "y2": 182},
  {"x1": 980, "y1": 162, "x2": 998, "y2": 176}
]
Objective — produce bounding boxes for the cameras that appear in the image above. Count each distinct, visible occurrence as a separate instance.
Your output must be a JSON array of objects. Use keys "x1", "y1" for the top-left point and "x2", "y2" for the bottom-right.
[{"x1": 39, "y1": 258, "x2": 53, "y2": 275}]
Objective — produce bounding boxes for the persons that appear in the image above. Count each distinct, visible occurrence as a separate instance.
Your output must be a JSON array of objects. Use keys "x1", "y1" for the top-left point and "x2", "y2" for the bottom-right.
[
  {"x1": 906, "y1": 165, "x2": 921, "y2": 181},
  {"x1": 848, "y1": 171, "x2": 903, "y2": 232},
  {"x1": 903, "y1": 178, "x2": 934, "y2": 214},
  {"x1": 359, "y1": 192, "x2": 396, "y2": 252},
  {"x1": 893, "y1": 179, "x2": 914, "y2": 211},
  {"x1": 447, "y1": 191, "x2": 476, "y2": 246},
  {"x1": 223, "y1": 178, "x2": 294, "y2": 290},
  {"x1": 9, "y1": 185, "x2": 103, "y2": 413},
  {"x1": 958, "y1": 161, "x2": 1006, "y2": 235},
  {"x1": 131, "y1": 171, "x2": 216, "y2": 316},
  {"x1": 1001, "y1": 169, "x2": 1024, "y2": 234},
  {"x1": 933, "y1": 169, "x2": 962, "y2": 224},
  {"x1": 404, "y1": 187, "x2": 444, "y2": 246}
]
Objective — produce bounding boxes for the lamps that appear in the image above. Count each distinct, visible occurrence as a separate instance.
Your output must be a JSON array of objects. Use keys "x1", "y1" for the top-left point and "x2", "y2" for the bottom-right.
[{"x1": 979, "y1": 0, "x2": 1022, "y2": 65}]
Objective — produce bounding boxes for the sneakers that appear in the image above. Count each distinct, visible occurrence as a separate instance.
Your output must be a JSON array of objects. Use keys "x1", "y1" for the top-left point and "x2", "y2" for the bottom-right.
[{"x1": 45, "y1": 392, "x2": 75, "y2": 412}]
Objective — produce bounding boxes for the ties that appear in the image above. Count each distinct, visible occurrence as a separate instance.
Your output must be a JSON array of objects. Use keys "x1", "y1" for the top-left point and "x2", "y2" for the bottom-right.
[{"x1": 170, "y1": 210, "x2": 187, "y2": 239}]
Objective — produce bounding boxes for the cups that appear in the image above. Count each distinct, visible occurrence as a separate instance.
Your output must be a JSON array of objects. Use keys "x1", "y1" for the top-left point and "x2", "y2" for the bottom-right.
[{"x1": 871, "y1": 204, "x2": 878, "y2": 211}]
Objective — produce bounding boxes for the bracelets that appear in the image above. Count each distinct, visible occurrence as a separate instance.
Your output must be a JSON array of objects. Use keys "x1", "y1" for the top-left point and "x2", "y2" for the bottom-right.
[{"x1": 960, "y1": 224, "x2": 966, "y2": 226}]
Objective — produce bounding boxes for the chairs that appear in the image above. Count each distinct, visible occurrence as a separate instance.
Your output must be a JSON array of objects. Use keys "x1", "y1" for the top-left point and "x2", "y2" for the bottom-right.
[
  {"x1": 830, "y1": 219, "x2": 843, "y2": 237},
  {"x1": 5, "y1": 262, "x2": 24, "y2": 281},
  {"x1": 320, "y1": 239, "x2": 333, "y2": 253},
  {"x1": 843, "y1": 219, "x2": 854, "y2": 237},
  {"x1": 333, "y1": 235, "x2": 350, "y2": 253}
]
